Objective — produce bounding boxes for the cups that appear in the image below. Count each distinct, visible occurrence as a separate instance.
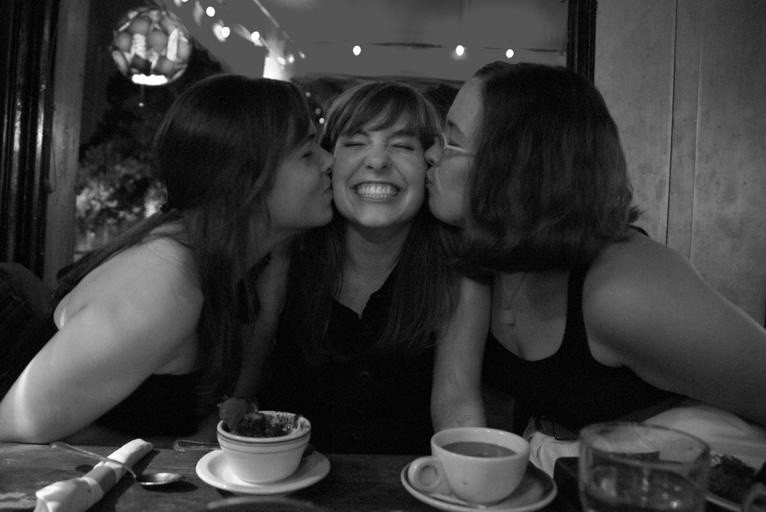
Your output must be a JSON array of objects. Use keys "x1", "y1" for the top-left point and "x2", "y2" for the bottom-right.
[
  {"x1": 577, "y1": 421, "x2": 714, "y2": 511},
  {"x1": 399, "y1": 460, "x2": 557, "y2": 507},
  {"x1": 216, "y1": 410, "x2": 311, "y2": 483},
  {"x1": 407, "y1": 425, "x2": 531, "y2": 504}
]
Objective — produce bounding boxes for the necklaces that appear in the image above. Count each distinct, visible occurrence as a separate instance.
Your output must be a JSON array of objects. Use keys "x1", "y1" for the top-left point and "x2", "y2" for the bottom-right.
[{"x1": 497, "y1": 272, "x2": 527, "y2": 326}]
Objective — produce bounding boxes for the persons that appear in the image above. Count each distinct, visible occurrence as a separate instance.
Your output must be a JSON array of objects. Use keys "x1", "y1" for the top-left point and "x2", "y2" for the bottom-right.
[
  {"x1": 299, "y1": 80, "x2": 461, "y2": 456},
  {"x1": 424, "y1": 62, "x2": 766, "y2": 447},
  {"x1": 0, "y1": 75, "x2": 335, "y2": 445}
]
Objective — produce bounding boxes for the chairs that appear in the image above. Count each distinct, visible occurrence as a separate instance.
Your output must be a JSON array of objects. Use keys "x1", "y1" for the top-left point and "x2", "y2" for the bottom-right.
[{"x1": 0, "y1": 262, "x2": 53, "y2": 382}]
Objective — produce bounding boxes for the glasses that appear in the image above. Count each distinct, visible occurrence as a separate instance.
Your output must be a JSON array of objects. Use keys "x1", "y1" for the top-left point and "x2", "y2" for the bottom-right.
[{"x1": 438, "y1": 128, "x2": 479, "y2": 157}]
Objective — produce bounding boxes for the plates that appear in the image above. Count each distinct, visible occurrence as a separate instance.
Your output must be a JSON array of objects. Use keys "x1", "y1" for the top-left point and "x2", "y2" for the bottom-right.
[{"x1": 195, "y1": 448, "x2": 331, "y2": 497}]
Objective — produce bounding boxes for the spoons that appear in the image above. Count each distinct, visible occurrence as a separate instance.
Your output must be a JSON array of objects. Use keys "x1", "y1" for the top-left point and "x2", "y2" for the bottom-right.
[{"x1": 48, "y1": 440, "x2": 185, "y2": 491}]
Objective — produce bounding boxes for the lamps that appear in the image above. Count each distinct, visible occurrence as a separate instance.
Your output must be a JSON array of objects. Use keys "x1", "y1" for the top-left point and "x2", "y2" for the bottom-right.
[{"x1": 111, "y1": 0, "x2": 192, "y2": 87}]
